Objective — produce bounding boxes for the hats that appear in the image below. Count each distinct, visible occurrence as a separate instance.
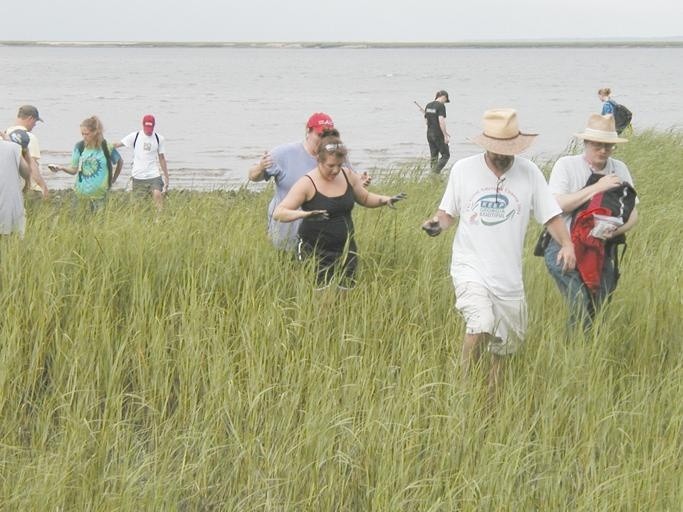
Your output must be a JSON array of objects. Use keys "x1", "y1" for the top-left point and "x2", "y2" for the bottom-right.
[
  {"x1": 471, "y1": 109, "x2": 538, "y2": 156},
  {"x1": 439, "y1": 91, "x2": 450, "y2": 104},
  {"x1": 10, "y1": 129, "x2": 29, "y2": 148},
  {"x1": 307, "y1": 113, "x2": 333, "y2": 134},
  {"x1": 144, "y1": 115, "x2": 154, "y2": 135},
  {"x1": 573, "y1": 114, "x2": 628, "y2": 143},
  {"x1": 18, "y1": 105, "x2": 44, "y2": 122}
]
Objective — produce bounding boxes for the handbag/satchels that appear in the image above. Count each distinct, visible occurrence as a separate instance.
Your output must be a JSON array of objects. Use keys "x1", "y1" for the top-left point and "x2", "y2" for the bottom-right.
[
  {"x1": 534, "y1": 230, "x2": 550, "y2": 257},
  {"x1": 610, "y1": 102, "x2": 632, "y2": 133},
  {"x1": 572, "y1": 173, "x2": 636, "y2": 245}
]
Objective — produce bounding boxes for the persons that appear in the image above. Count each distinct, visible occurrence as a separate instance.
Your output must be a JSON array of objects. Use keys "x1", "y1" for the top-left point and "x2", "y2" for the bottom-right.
[
  {"x1": 424, "y1": 90, "x2": 450, "y2": 174},
  {"x1": 424, "y1": 108, "x2": 576, "y2": 411},
  {"x1": 0, "y1": 130, "x2": 32, "y2": 239},
  {"x1": 113, "y1": 115, "x2": 168, "y2": 224},
  {"x1": 273, "y1": 127, "x2": 407, "y2": 306},
  {"x1": 544, "y1": 114, "x2": 640, "y2": 337},
  {"x1": 249, "y1": 112, "x2": 373, "y2": 251},
  {"x1": 598, "y1": 88, "x2": 625, "y2": 151},
  {"x1": 48, "y1": 115, "x2": 124, "y2": 224},
  {"x1": 5, "y1": 105, "x2": 50, "y2": 202}
]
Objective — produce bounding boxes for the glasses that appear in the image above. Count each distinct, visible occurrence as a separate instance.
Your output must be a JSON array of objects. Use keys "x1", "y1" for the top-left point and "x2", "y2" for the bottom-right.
[
  {"x1": 320, "y1": 143, "x2": 347, "y2": 154},
  {"x1": 592, "y1": 142, "x2": 617, "y2": 152}
]
